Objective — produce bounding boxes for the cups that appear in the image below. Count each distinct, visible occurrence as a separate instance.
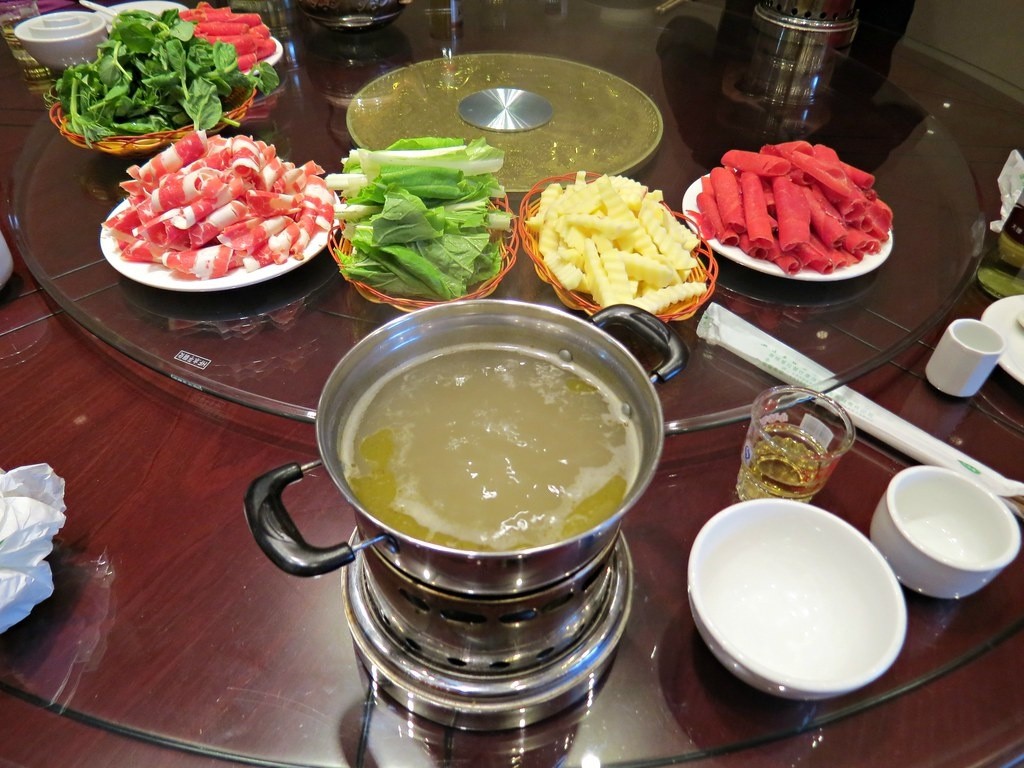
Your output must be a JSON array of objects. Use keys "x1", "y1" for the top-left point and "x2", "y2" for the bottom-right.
[
  {"x1": 737, "y1": 384, "x2": 857, "y2": 505},
  {"x1": 925, "y1": 318, "x2": 1005, "y2": 397},
  {"x1": 43, "y1": 13, "x2": 80, "y2": 28},
  {"x1": 869, "y1": 464, "x2": 1022, "y2": 597},
  {"x1": 0, "y1": 230, "x2": 13, "y2": 288}
]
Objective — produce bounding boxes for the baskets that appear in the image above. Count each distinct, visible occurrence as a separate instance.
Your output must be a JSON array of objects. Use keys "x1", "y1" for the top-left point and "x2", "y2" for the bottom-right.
[
  {"x1": 518, "y1": 171, "x2": 718, "y2": 323},
  {"x1": 327, "y1": 190, "x2": 519, "y2": 312},
  {"x1": 48, "y1": 78, "x2": 256, "y2": 156}
]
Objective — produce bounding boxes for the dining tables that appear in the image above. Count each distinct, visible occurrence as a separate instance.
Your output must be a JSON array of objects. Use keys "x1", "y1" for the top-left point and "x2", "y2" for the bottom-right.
[{"x1": 1, "y1": 0, "x2": 1024, "y2": 768}]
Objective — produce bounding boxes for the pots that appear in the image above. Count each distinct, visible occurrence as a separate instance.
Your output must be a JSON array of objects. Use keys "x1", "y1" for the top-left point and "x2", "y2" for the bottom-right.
[
  {"x1": 710, "y1": 0, "x2": 860, "y2": 109},
  {"x1": 241, "y1": 299, "x2": 692, "y2": 598},
  {"x1": 295, "y1": 0, "x2": 411, "y2": 32}
]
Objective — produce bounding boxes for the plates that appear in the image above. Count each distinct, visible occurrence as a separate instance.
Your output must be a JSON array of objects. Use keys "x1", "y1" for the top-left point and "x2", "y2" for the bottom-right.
[
  {"x1": 244, "y1": 35, "x2": 284, "y2": 77},
  {"x1": 99, "y1": 192, "x2": 342, "y2": 293},
  {"x1": 94, "y1": 1, "x2": 190, "y2": 33},
  {"x1": 686, "y1": 498, "x2": 907, "y2": 700},
  {"x1": 682, "y1": 171, "x2": 895, "y2": 280},
  {"x1": 981, "y1": 292, "x2": 1024, "y2": 385}
]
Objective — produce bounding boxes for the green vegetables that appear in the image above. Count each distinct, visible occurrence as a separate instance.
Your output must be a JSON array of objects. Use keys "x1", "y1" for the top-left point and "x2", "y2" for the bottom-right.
[
  {"x1": 52, "y1": 6, "x2": 279, "y2": 149},
  {"x1": 325, "y1": 136, "x2": 514, "y2": 304}
]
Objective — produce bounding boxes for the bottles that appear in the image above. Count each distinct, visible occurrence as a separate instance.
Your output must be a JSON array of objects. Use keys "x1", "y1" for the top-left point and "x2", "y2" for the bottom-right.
[
  {"x1": 976, "y1": 189, "x2": 1024, "y2": 298},
  {"x1": 0, "y1": 0, "x2": 54, "y2": 79}
]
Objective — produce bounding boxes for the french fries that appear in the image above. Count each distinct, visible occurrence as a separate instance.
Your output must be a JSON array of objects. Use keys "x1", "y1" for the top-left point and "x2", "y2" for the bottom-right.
[{"x1": 523, "y1": 168, "x2": 708, "y2": 313}]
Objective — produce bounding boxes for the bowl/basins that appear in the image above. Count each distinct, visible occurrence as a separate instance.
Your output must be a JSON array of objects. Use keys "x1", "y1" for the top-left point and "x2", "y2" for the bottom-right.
[
  {"x1": 27, "y1": 16, "x2": 94, "y2": 40},
  {"x1": 14, "y1": 11, "x2": 107, "y2": 73}
]
